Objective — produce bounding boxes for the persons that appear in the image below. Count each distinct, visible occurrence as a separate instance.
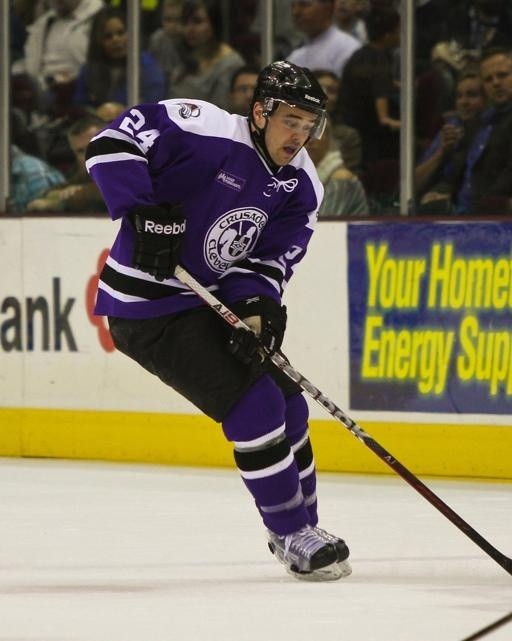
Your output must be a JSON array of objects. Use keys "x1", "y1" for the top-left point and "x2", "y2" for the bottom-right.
[{"x1": 82, "y1": 61, "x2": 350, "y2": 572}]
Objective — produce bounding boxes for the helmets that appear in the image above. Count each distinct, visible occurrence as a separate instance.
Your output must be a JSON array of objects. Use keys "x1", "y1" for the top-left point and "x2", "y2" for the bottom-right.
[{"x1": 249, "y1": 59, "x2": 327, "y2": 168}]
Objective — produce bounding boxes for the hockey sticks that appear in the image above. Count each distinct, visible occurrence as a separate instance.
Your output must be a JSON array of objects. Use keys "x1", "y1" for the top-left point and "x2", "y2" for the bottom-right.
[{"x1": 174, "y1": 266, "x2": 512, "y2": 576}]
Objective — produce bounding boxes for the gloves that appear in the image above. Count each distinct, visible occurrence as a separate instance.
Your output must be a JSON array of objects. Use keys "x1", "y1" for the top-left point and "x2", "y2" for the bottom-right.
[
  {"x1": 131, "y1": 202, "x2": 189, "y2": 284},
  {"x1": 220, "y1": 293, "x2": 287, "y2": 367}
]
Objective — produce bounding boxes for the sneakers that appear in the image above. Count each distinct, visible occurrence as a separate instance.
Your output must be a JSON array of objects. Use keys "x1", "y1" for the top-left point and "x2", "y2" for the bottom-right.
[{"x1": 267, "y1": 524, "x2": 349, "y2": 574}]
